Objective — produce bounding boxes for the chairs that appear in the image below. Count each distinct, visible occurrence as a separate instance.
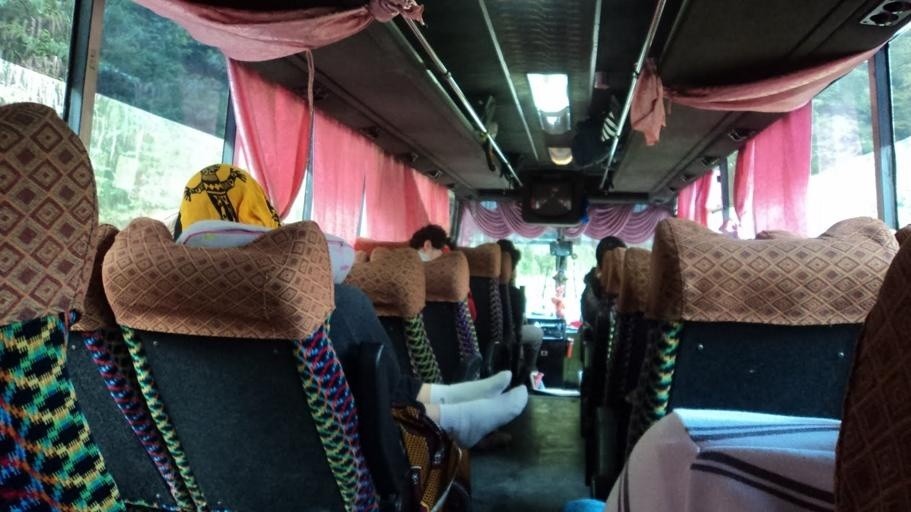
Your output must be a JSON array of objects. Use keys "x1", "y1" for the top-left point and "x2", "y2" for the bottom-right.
[
  {"x1": 0, "y1": 100, "x2": 198, "y2": 511},
  {"x1": 348, "y1": 243, "x2": 526, "y2": 501},
  {"x1": 65, "y1": 223, "x2": 195, "y2": 511},
  {"x1": 582, "y1": 216, "x2": 911, "y2": 512},
  {"x1": 99, "y1": 216, "x2": 471, "y2": 512}
]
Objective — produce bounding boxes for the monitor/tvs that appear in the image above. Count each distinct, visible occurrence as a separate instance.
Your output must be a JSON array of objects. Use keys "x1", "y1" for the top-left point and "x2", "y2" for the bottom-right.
[{"x1": 521, "y1": 169, "x2": 585, "y2": 224}]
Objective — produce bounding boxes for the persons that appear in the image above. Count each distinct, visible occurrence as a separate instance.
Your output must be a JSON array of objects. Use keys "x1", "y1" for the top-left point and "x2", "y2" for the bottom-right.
[
  {"x1": 580, "y1": 236, "x2": 628, "y2": 328},
  {"x1": 179, "y1": 164, "x2": 471, "y2": 512},
  {"x1": 392, "y1": 370, "x2": 529, "y2": 449},
  {"x1": 601, "y1": 408, "x2": 842, "y2": 512},
  {"x1": 519, "y1": 324, "x2": 543, "y2": 370},
  {"x1": 497, "y1": 239, "x2": 526, "y2": 341},
  {"x1": 410, "y1": 224, "x2": 477, "y2": 322}
]
